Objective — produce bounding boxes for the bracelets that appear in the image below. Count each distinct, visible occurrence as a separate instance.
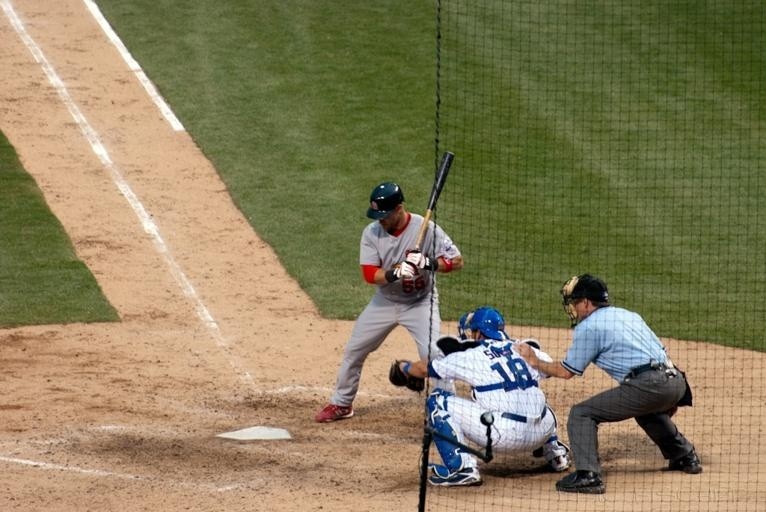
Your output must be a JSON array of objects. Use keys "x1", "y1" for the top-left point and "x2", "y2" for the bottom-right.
[{"x1": 403, "y1": 363, "x2": 411, "y2": 373}]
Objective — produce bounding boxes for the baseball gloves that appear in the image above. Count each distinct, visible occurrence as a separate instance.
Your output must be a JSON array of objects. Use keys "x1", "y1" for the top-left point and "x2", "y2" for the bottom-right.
[{"x1": 390, "y1": 360, "x2": 424, "y2": 391}]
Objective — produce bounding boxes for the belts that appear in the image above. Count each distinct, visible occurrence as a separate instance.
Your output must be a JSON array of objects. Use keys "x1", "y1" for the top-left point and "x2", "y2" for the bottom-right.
[
  {"x1": 624, "y1": 363, "x2": 667, "y2": 381},
  {"x1": 501, "y1": 405, "x2": 546, "y2": 423}
]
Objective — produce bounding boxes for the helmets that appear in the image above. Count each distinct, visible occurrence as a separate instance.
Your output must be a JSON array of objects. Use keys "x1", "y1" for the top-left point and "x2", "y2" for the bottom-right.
[
  {"x1": 457, "y1": 307, "x2": 510, "y2": 341},
  {"x1": 559, "y1": 274, "x2": 611, "y2": 328},
  {"x1": 366, "y1": 182, "x2": 404, "y2": 220}
]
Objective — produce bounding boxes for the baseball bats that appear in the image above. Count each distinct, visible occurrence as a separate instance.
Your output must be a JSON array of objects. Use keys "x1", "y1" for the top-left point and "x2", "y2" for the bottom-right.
[{"x1": 413, "y1": 152, "x2": 454, "y2": 254}]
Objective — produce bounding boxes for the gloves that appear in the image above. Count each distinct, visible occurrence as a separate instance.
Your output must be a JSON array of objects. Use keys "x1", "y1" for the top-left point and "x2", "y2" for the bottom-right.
[{"x1": 384, "y1": 249, "x2": 438, "y2": 283}]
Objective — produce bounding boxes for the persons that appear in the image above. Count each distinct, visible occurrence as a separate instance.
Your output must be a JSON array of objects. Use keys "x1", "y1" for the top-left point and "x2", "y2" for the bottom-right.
[
  {"x1": 389, "y1": 305, "x2": 572, "y2": 487},
  {"x1": 511, "y1": 272, "x2": 703, "y2": 493},
  {"x1": 313, "y1": 179, "x2": 465, "y2": 424}
]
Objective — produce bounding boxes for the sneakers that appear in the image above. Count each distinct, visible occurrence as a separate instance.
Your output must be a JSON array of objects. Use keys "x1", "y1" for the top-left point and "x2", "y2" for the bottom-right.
[
  {"x1": 316, "y1": 404, "x2": 354, "y2": 423},
  {"x1": 427, "y1": 467, "x2": 482, "y2": 487},
  {"x1": 547, "y1": 454, "x2": 572, "y2": 472},
  {"x1": 669, "y1": 447, "x2": 702, "y2": 474},
  {"x1": 556, "y1": 471, "x2": 605, "y2": 493}
]
